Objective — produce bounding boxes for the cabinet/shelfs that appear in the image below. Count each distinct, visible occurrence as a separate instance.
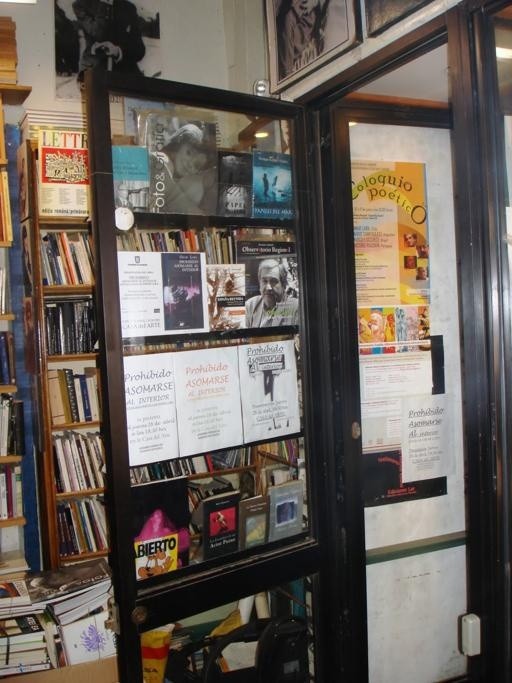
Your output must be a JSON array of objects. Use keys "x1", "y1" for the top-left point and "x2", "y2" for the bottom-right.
[
  {"x1": 16, "y1": 138, "x2": 302, "y2": 572},
  {"x1": 0, "y1": 84, "x2": 32, "y2": 575}
]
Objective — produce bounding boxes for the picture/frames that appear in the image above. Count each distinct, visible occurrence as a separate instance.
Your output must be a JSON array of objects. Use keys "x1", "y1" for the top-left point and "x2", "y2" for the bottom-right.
[
  {"x1": 365, "y1": 0, "x2": 432, "y2": 38},
  {"x1": 263, "y1": 0, "x2": 362, "y2": 95}
]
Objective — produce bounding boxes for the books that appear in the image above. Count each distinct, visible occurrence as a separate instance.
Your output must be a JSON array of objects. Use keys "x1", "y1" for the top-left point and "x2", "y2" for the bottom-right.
[
  {"x1": 132, "y1": 464, "x2": 306, "y2": 582},
  {"x1": 110, "y1": 108, "x2": 296, "y2": 219},
  {"x1": 0, "y1": 14, "x2": 17, "y2": 84},
  {"x1": 1, "y1": 109, "x2": 116, "y2": 674},
  {"x1": 111, "y1": 227, "x2": 300, "y2": 337},
  {"x1": 122, "y1": 334, "x2": 302, "y2": 484}
]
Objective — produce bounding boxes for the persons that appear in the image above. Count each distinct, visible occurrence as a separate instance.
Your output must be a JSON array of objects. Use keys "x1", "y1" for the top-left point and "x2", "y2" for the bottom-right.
[
  {"x1": 73, "y1": 0, "x2": 147, "y2": 86},
  {"x1": 357, "y1": 306, "x2": 431, "y2": 354},
  {"x1": 403, "y1": 232, "x2": 417, "y2": 245},
  {"x1": 415, "y1": 243, "x2": 428, "y2": 257},
  {"x1": 404, "y1": 255, "x2": 417, "y2": 269},
  {"x1": 415, "y1": 266, "x2": 428, "y2": 279}
]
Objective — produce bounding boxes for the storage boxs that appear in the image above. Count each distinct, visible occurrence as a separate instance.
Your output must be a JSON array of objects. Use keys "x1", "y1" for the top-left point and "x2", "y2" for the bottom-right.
[{"x1": 0, "y1": 654, "x2": 119, "y2": 683}]
